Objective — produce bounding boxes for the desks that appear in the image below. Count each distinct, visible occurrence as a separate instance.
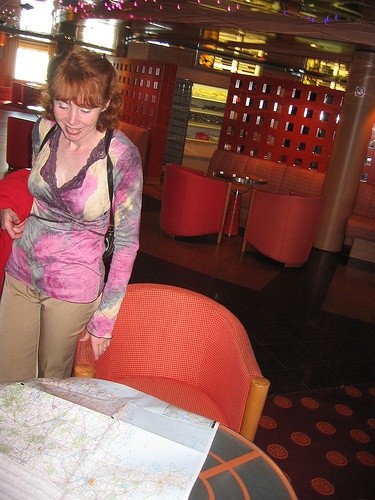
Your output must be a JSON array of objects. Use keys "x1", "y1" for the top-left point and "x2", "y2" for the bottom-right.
[
  {"x1": 213, "y1": 169, "x2": 268, "y2": 236},
  {"x1": 188, "y1": 425, "x2": 298, "y2": 500}
]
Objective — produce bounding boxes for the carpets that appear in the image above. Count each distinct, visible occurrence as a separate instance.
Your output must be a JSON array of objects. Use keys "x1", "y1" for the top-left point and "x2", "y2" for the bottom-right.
[
  {"x1": 138, "y1": 210, "x2": 285, "y2": 290},
  {"x1": 252, "y1": 383, "x2": 375, "y2": 500},
  {"x1": 320, "y1": 263, "x2": 375, "y2": 323}
]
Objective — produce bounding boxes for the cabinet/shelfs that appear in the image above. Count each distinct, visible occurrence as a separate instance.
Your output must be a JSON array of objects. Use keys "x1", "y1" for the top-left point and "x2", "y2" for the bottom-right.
[
  {"x1": 345, "y1": 182, "x2": 375, "y2": 263},
  {"x1": 183, "y1": 82, "x2": 229, "y2": 160}
]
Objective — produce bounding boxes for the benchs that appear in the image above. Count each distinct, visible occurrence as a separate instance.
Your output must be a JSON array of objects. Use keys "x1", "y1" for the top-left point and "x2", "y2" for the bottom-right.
[
  {"x1": 118, "y1": 122, "x2": 149, "y2": 170},
  {"x1": 205, "y1": 149, "x2": 325, "y2": 234}
]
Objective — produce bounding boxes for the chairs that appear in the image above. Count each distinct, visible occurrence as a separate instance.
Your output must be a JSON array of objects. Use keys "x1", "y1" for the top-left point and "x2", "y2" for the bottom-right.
[
  {"x1": 73, "y1": 283, "x2": 270, "y2": 444},
  {"x1": 159, "y1": 164, "x2": 228, "y2": 241},
  {"x1": 6, "y1": 117, "x2": 35, "y2": 171},
  {"x1": 241, "y1": 189, "x2": 325, "y2": 267}
]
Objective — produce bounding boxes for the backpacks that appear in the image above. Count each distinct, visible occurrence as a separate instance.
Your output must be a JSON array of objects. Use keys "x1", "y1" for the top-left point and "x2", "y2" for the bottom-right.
[{"x1": 39, "y1": 124, "x2": 115, "y2": 283}]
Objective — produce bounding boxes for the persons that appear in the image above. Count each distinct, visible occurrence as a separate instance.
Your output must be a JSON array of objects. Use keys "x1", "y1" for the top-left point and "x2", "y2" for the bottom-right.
[
  {"x1": 32, "y1": 48, "x2": 69, "y2": 169},
  {"x1": 0, "y1": 47, "x2": 143, "y2": 382}
]
